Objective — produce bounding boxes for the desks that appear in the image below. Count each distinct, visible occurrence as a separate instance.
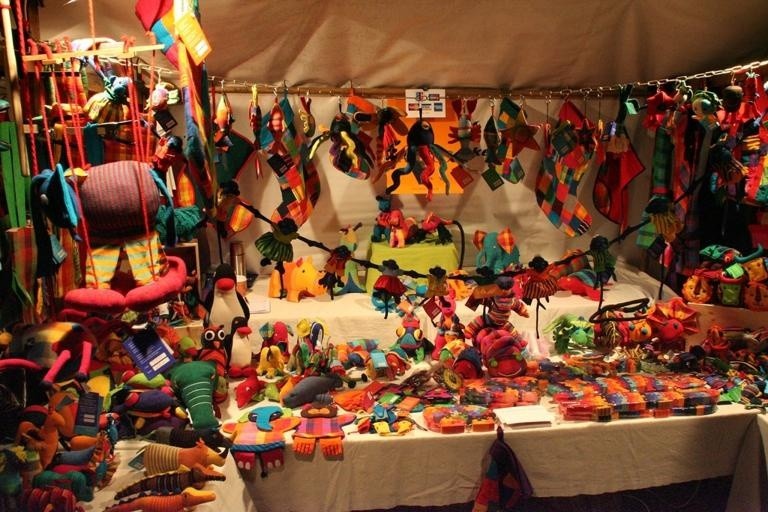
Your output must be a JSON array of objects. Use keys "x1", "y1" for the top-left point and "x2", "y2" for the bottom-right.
[{"x1": 76, "y1": 351, "x2": 768, "y2": 512}]
